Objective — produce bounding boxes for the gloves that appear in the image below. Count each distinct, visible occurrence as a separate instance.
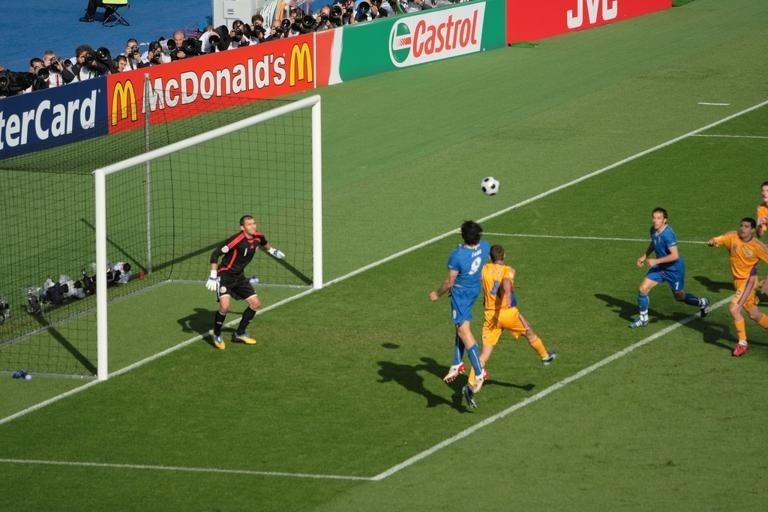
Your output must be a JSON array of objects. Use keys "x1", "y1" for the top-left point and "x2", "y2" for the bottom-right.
[
  {"x1": 267, "y1": 246, "x2": 286, "y2": 259},
  {"x1": 205, "y1": 270, "x2": 218, "y2": 291}
]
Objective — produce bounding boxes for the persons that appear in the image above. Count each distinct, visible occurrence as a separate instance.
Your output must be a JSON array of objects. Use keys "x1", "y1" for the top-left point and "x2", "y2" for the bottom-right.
[
  {"x1": 706, "y1": 217, "x2": 767, "y2": 358},
  {"x1": 459, "y1": 244, "x2": 558, "y2": 409},
  {"x1": 627, "y1": 207, "x2": 711, "y2": 328},
  {"x1": 0, "y1": 0, "x2": 479, "y2": 96},
  {"x1": 205, "y1": 215, "x2": 286, "y2": 351},
  {"x1": 427, "y1": 220, "x2": 494, "y2": 395},
  {"x1": 751, "y1": 182, "x2": 767, "y2": 301}
]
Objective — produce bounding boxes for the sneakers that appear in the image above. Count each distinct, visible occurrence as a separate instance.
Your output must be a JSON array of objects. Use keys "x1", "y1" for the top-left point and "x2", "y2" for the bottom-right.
[
  {"x1": 213, "y1": 335, "x2": 225, "y2": 350},
  {"x1": 700, "y1": 297, "x2": 709, "y2": 317},
  {"x1": 462, "y1": 386, "x2": 477, "y2": 410},
  {"x1": 472, "y1": 369, "x2": 489, "y2": 393},
  {"x1": 443, "y1": 362, "x2": 465, "y2": 384},
  {"x1": 733, "y1": 341, "x2": 748, "y2": 355},
  {"x1": 231, "y1": 332, "x2": 257, "y2": 345},
  {"x1": 541, "y1": 352, "x2": 556, "y2": 365},
  {"x1": 628, "y1": 320, "x2": 648, "y2": 327}
]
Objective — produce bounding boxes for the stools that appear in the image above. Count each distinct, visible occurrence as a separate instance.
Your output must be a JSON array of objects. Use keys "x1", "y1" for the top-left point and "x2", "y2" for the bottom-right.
[{"x1": 97, "y1": 0, "x2": 130, "y2": 28}]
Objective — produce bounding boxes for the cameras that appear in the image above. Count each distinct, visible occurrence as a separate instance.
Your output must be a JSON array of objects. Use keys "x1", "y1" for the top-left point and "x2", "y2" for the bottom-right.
[
  {"x1": 167, "y1": 38, "x2": 179, "y2": 61},
  {"x1": 97, "y1": 47, "x2": 110, "y2": 62},
  {"x1": 183, "y1": 37, "x2": 201, "y2": 53},
  {"x1": 304, "y1": 15, "x2": 316, "y2": 27},
  {"x1": 355, "y1": 2, "x2": 369, "y2": 22},
  {"x1": 50, "y1": 57, "x2": 58, "y2": 66},
  {"x1": 276, "y1": 19, "x2": 290, "y2": 36},
  {"x1": 1, "y1": 69, "x2": 34, "y2": 94},
  {"x1": 343, "y1": 8, "x2": 352, "y2": 25},
  {"x1": 232, "y1": 27, "x2": 244, "y2": 42},
  {"x1": 321, "y1": 14, "x2": 328, "y2": 23},
  {"x1": 85, "y1": 51, "x2": 93, "y2": 63},
  {"x1": 64, "y1": 59, "x2": 71, "y2": 64},
  {"x1": 255, "y1": 24, "x2": 266, "y2": 37},
  {"x1": 153, "y1": 52, "x2": 160, "y2": 61},
  {"x1": 132, "y1": 46, "x2": 138, "y2": 55},
  {"x1": 36, "y1": 67, "x2": 49, "y2": 80},
  {"x1": 331, "y1": 5, "x2": 340, "y2": 24}
]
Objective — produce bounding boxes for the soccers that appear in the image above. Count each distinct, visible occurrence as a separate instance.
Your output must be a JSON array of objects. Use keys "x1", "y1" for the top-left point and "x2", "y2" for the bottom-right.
[{"x1": 481, "y1": 177, "x2": 500, "y2": 196}]
